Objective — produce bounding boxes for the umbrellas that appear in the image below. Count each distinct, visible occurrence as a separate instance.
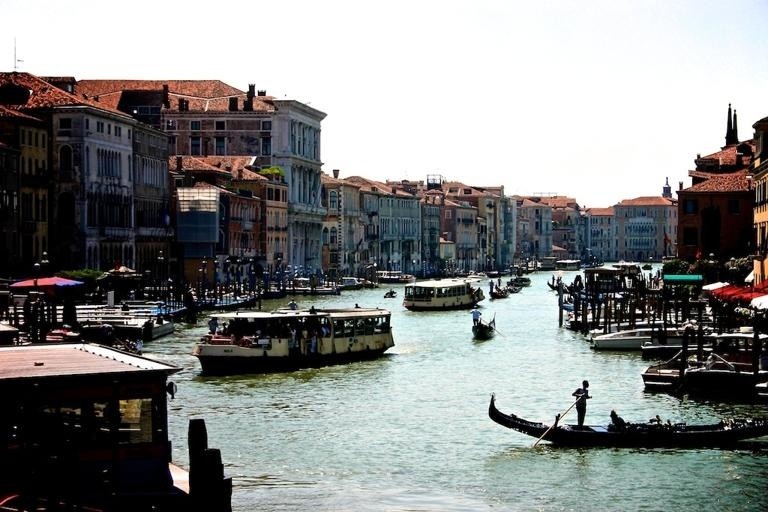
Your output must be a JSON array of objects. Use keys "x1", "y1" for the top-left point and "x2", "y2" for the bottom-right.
[
  {"x1": 13, "y1": 276, "x2": 85, "y2": 288},
  {"x1": 702, "y1": 279, "x2": 767, "y2": 312},
  {"x1": 109, "y1": 265, "x2": 136, "y2": 278}
]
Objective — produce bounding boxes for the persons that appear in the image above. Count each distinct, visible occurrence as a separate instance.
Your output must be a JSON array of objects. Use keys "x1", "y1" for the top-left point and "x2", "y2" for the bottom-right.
[
  {"x1": 497, "y1": 276, "x2": 501, "y2": 287},
  {"x1": 287, "y1": 299, "x2": 298, "y2": 309},
  {"x1": 572, "y1": 379, "x2": 593, "y2": 430},
  {"x1": 208, "y1": 317, "x2": 218, "y2": 334},
  {"x1": 469, "y1": 305, "x2": 482, "y2": 326},
  {"x1": 124, "y1": 338, "x2": 142, "y2": 356},
  {"x1": 289, "y1": 327, "x2": 297, "y2": 341},
  {"x1": 221, "y1": 321, "x2": 227, "y2": 334},
  {"x1": 308, "y1": 305, "x2": 316, "y2": 315},
  {"x1": 488, "y1": 279, "x2": 494, "y2": 292}
]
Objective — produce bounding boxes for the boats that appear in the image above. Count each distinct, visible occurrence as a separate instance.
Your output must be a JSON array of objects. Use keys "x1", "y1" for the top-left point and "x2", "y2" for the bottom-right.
[
  {"x1": 190, "y1": 296, "x2": 396, "y2": 377},
  {"x1": 592, "y1": 327, "x2": 685, "y2": 350},
  {"x1": 402, "y1": 278, "x2": 486, "y2": 310},
  {"x1": 488, "y1": 276, "x2": 532, "y2": 299},
  {"x1": 486, "y1": 391, "x2": 768, "y2": 447},
  {"x1": 472, "y1": 318, "x2": 496, "y2": 341}
]
